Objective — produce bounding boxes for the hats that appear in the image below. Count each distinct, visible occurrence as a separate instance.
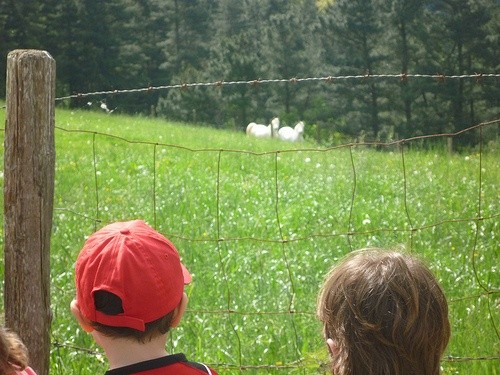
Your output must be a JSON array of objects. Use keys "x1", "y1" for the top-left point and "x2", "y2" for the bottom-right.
[{"x1": 74, "y1": 220, "x2": 192, "y2": 332}]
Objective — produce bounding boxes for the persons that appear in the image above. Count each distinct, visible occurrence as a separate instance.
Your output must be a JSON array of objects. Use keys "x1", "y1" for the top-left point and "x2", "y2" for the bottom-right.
[
  {"x1": 0, "y1": 320, "x2": 38, "y2": 374},
  {"x1": 68, "y1": 217, "x2": 223, "y2": 375},
  {"x1": 315, "y1": 244, "x2": 451, "y2": 375}
]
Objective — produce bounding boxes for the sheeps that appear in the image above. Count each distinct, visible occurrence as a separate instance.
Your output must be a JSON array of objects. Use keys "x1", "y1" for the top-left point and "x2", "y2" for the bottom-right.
[
  {"x1": 279, "y1": 121, "x2": 305, "y2": 142},
  {"x1": 246, "y1": 118, "x2": 280, "y2": 139}
]
